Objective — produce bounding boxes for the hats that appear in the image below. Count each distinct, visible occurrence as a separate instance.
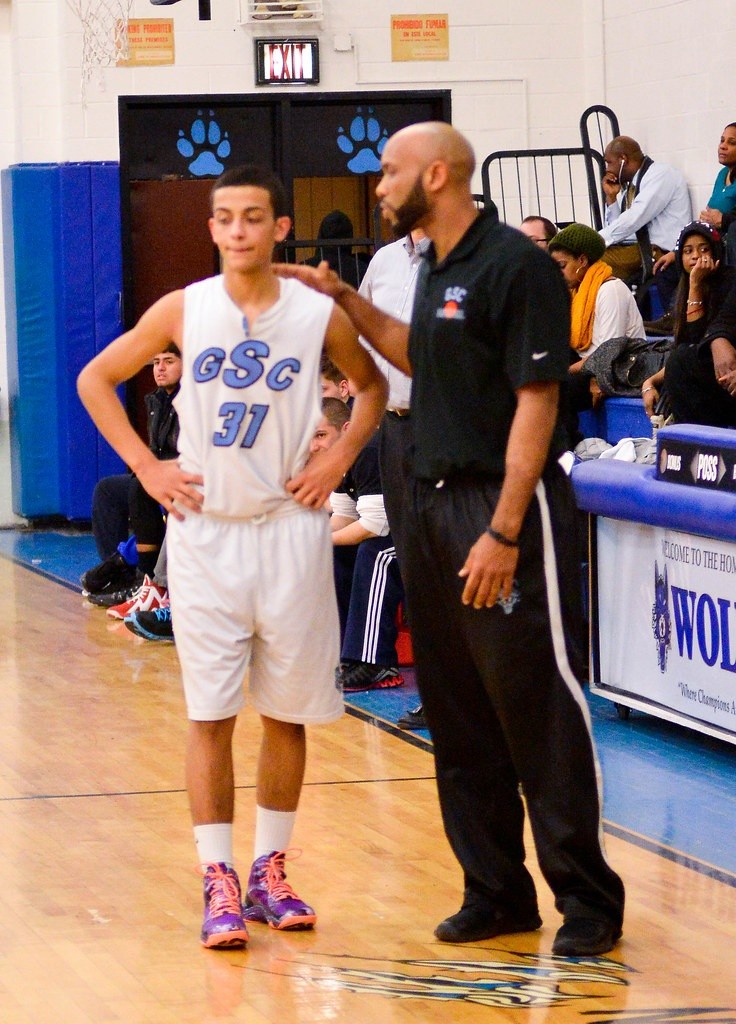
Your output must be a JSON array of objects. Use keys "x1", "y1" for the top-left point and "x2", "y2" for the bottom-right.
[
  {"x1": 548, "y1": 223, "x2": 606, "y2": 265},
  {"x1": 675, "y1": 221, "x2": 721, "y2": 259}
]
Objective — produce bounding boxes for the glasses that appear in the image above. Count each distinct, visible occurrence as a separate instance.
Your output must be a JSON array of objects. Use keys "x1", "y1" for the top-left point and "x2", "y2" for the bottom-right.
[{"x1": 531, "y1": 239, "x2": 547, "y2": 244}]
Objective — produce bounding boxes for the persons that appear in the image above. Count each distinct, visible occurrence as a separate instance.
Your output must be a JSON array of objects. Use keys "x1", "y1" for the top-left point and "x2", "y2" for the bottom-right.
[
  {"x1": 77, "y1": 122, "x2": 736, "y2": 730},
  {"x1": 76, "y1": 163, "x2": 389, "y2": 950},
  {"x1": 266, "y1": 121, "x2": 622, "y2": 958}
]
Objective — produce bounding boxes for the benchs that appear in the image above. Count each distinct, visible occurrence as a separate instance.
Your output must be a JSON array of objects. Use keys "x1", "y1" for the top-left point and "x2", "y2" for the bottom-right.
[{"x1": 578, "y1": 281, "x2": 662, "y2": 444}]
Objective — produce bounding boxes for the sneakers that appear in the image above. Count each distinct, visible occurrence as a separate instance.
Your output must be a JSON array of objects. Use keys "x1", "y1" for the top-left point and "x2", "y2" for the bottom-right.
[
  {"x1": 435, "y1": 908, "x2": 543, "y2": 943},
  {"x1": 336, "y1": 660, "x2": 405, "y2": 692},
  {"x1": 195, "y1": 861, "x2": 250, "y2": 951},
  {"x1": 397, "y1": 704, "x2": 428, "y2": 729},
  {"x1": 242, "y1": 847, "x2": 317, "y2": 930},
  {"x1": 552, "y1": 919, "x2": 623, "y2": 956},
  {"x1": 79, "y1": 551, "x2": 174, "y2": 641}
]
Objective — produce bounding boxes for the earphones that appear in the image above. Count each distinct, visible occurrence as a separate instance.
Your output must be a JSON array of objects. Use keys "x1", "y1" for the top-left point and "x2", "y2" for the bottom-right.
[{"x1": 622, "y1": 159, "x2": 625, "y2": 165}]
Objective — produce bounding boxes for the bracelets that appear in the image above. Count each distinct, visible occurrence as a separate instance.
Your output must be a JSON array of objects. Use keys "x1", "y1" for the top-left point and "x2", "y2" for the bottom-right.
[
  {"x1": 686, "y1": 307, "x2": 703, "y2": 315},
  {"x1": 485, "y1": 526, "x2": 517, "y2": 547},
  {"x1": 641, "y1": 386, "x2": 655, "y2": 395},
  {"x1": 686, "y1": 300, "x2": 703, "y2": 305}
]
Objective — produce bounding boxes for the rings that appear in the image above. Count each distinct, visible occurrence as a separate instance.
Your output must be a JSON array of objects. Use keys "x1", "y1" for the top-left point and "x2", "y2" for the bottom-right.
[{"x1": 701, "y1": 259, "x2": 707, "y2": 262}]
